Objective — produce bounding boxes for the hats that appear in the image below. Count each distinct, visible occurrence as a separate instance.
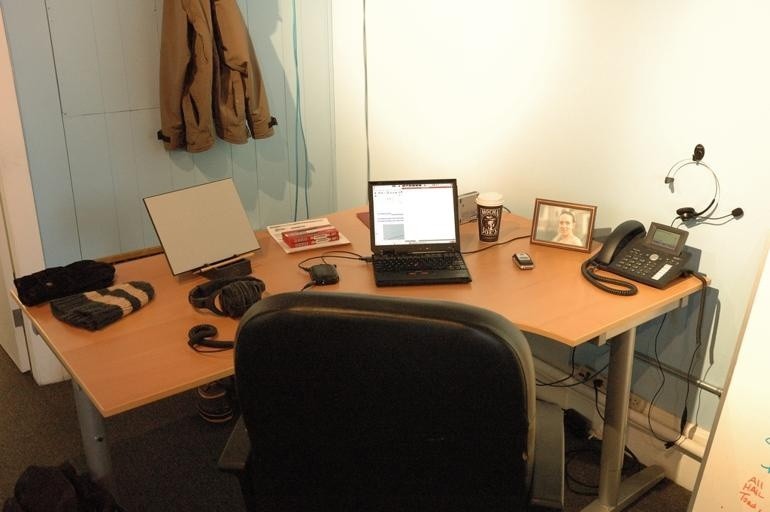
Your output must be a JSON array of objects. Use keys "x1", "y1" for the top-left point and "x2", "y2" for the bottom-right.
[{"x1": 50, "y1": 280, "x2": 155, "y2": 332}]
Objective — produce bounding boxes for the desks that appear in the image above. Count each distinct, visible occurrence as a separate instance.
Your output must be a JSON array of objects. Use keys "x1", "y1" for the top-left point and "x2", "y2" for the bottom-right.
[{"x1": 10, "y1": 206, "x2": 713, "y2": 512}]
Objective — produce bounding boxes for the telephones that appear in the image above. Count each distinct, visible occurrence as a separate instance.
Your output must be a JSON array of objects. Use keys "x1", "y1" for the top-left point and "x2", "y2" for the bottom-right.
[{"x1": 593, "y1": 220, "x2": 692, "y2": 288}]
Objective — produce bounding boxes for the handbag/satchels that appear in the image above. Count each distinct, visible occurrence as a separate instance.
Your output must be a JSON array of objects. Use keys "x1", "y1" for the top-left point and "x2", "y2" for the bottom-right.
[{"x1": 1, "y1": 463, "x2": 122, "y2": 512}]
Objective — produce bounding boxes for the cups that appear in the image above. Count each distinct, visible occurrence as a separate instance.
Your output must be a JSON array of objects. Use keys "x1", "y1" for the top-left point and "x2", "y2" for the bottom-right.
[{"x1": 475, "y1": 191, "x2": 504, "y2": 242}]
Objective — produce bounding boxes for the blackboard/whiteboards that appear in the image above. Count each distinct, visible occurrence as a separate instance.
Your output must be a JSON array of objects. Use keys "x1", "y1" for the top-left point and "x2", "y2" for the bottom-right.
[{"x1": 686, "y1": 231, "x2": 770, "y2": 512}]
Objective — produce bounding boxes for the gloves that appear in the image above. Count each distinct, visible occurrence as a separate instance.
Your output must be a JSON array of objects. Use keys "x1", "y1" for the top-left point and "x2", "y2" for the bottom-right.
[{"x1": 13, "y1": 260, "x2": 115, "y2": 307}]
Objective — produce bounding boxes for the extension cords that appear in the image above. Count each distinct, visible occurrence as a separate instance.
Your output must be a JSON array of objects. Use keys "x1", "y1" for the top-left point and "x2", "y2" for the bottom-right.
[{"x1": 576, "y1": 364, "x2": 646, "y2": 413}]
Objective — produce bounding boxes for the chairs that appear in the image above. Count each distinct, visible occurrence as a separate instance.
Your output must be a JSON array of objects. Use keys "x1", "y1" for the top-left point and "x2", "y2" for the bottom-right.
[{"x1": 218, "y1": 291, "x2": 567, "y2": 512}]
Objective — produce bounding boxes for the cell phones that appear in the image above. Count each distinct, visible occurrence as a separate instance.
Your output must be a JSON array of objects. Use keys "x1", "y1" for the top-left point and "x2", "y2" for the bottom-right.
[{"x1": 511, "y1": 252, "x2": 535, "y2": 270}]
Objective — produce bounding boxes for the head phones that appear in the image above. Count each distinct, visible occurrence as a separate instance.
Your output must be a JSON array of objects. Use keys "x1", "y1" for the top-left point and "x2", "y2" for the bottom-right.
[
  {"x1": 664, "y1": 158, "x2": 718, "y2": 221},
  {"x1": 188, "y1": 276, "x2": 266, "y2": 319}
]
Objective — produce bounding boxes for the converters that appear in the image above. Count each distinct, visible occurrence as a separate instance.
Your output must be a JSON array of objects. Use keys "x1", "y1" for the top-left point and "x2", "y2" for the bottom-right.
[{"x1": 309, "y1": 263, "x2": 340, "y2": 286}]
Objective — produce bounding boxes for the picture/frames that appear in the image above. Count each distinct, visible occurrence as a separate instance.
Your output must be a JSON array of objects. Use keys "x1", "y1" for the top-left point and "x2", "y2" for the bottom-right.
[{"x1": 530, "y1": 199, "x2": 596, "y2": 253}]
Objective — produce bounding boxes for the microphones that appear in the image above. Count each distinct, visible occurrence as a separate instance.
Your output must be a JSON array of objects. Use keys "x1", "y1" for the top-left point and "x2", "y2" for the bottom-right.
[{"x1": 698, "y1": 207, "x2": 742, "y2": 224}]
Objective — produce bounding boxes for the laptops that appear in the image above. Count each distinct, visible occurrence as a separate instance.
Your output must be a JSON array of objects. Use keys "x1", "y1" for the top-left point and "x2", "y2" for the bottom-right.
[{"x1": 368, "y1": 179, "x2": 472, "y2": 287}]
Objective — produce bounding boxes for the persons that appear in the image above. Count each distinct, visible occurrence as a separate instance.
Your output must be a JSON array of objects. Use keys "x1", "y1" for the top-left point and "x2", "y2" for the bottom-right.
[{"x1": 552, "y1": 211, "x2": 583, "y2": 247}]
